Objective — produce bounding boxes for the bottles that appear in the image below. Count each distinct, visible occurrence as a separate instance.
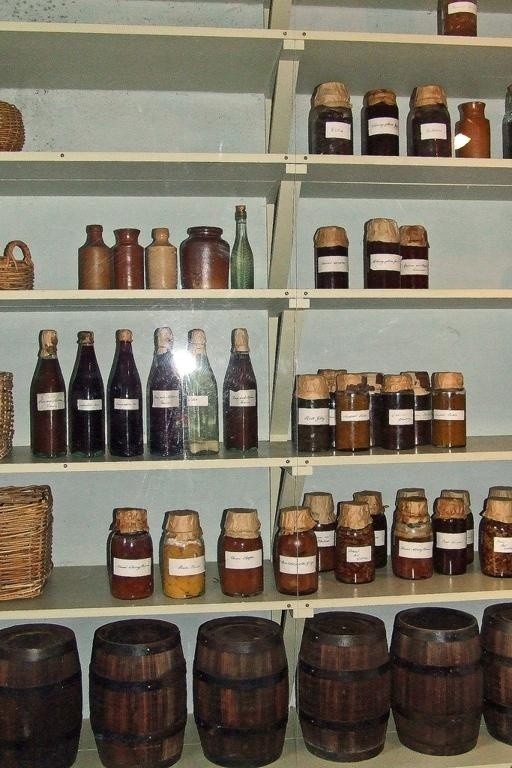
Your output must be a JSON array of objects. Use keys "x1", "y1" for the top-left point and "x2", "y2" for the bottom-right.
[
  {"x1": 453, "y1": 101, "x2": 492, "y2": 158},
  {"x1": 360, "y1": 89, "x2": 398, "y2": 155},
  {"x1": 503, "y1": 84, "x2": 512, "y2": 159},
  {"x1": 229, "y1": 203, "x2": 255, "y2": 289},
  {"x1": 184, "y1": 328, "x2": 220, "y2": 453},
  {"x1": 70, "y1": 330, "x2": 105, "y2": 457},
  {"x1": 76, "y1": 224, "x2": 113, "y2": 289},
  {"x1": 109, "y1": 227, "x2": 146, "y2": 289},
  {"x1": 289, "y1": 369, "x2": 467, "y2": 451},
  {"x1": 362, "y1": 219, "x2": 429, "y2": 289},
  {"x1": 108, "y1": 333, "x2": 143, "y2": 457},
  {"x1": 221, "y1": 327, "x2": 259, "y2": 450},
  {"x1": 335, "y1": 485, "x2": 511, "y2": 586},
  {"x1": 144, "y1": 227, "x2": 178, "y2": 289},
  {"x1": 29, "y1": 329, "x2": 64, "y2": 457},
  {"x1": 145, "y1": 326, "x2": 185, "y2": 455}
]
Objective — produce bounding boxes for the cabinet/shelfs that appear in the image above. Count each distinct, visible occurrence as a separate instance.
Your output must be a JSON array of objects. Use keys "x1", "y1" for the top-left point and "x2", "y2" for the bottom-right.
[{"x1": 0, "y1": 1, "x2": 512, "y2": 767}]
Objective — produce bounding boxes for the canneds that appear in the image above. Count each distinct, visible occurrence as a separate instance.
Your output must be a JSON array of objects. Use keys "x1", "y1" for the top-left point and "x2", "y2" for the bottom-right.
[
  {"x1": 398, "y1": 225, "x2": 430, "y2": 289},
  {"x1": 440, "y1": 490, "x2": 474, "y2": 565},
  {"x1": 291, "y1": 374, "x2": 331, "y2": 452},
  {"x1": 430, "y1": 497, "x2": 468, "y2": 575},
  {"x1": 391, "y1": 496, "x2": 434, "y2": 580},
  {"x1": 363, "y1": 372, "x2": 383, "y2": 447},
  {"x1": 431, "y1": 372, "x2": 465, "y2": 448},
  {"x1": 488, "y1": 486, "x2": 512, "y2": 504},
  {"x1": 367, "y1": 89, "x2": 399, "y2": 156},
  {"x1": 318, "y1": 369, "x2": 347, "y2": 449},
  {"x1": 363, "y1": 218, "x2": 401, "y2": 289},
  {"x1": 382, "y1": 372, "x2": 414, "y2": 450},
  {"x1": 334, "y1": 501, "x2": 376, "y2": 584},
  {"x1": 479, "y1": 497, "x2": 512, "y2": 578},
  {"x1": 335, "y1": 373, "x2": 370, "y2": 452},
  {"x1": 353, "y1": 490, "x2": 387, "y2": 569},
  {"x1": 391, "y1": 488, "x2": 425, "y2": 530},
  {"x1": 400, "y1": 372, "x2": 431, "y2": 446}
]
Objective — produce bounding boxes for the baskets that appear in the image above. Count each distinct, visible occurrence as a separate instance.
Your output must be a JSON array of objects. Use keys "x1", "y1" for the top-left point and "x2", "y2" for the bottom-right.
[
  {"x1": 1, "y1": 371, "x2": 14, "y2": 459},
  {"x1": 0, "y1": 98, "x2": 25, "y2": 152},
  {"x1": 0, "y1": 239, "x2": 33, "y2": 288},
  {"x1": 0, "y1": 484, "x2": 53, "y2": 599}
]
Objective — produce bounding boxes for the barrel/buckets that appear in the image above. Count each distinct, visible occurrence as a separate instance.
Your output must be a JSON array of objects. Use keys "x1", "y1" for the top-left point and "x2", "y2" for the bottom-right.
[
  {"x1": 390, "y1": 606, "x2": 484, "y2": 757},
  {"x1": 192, "y1": 615, "x2": 290, "y2": 768},
  {"x1": 88, "y1": 618, "x2": 188, "y2": 768},
  {"x1": 479, "y1": 601, "x2": 511, "y2": 746},
  {"x1": 0, "y1": 622, "x2": 84, "y2": 768},
  {"x1": 294, "y1": 610, "x2": 392, "y2": 764}
]
